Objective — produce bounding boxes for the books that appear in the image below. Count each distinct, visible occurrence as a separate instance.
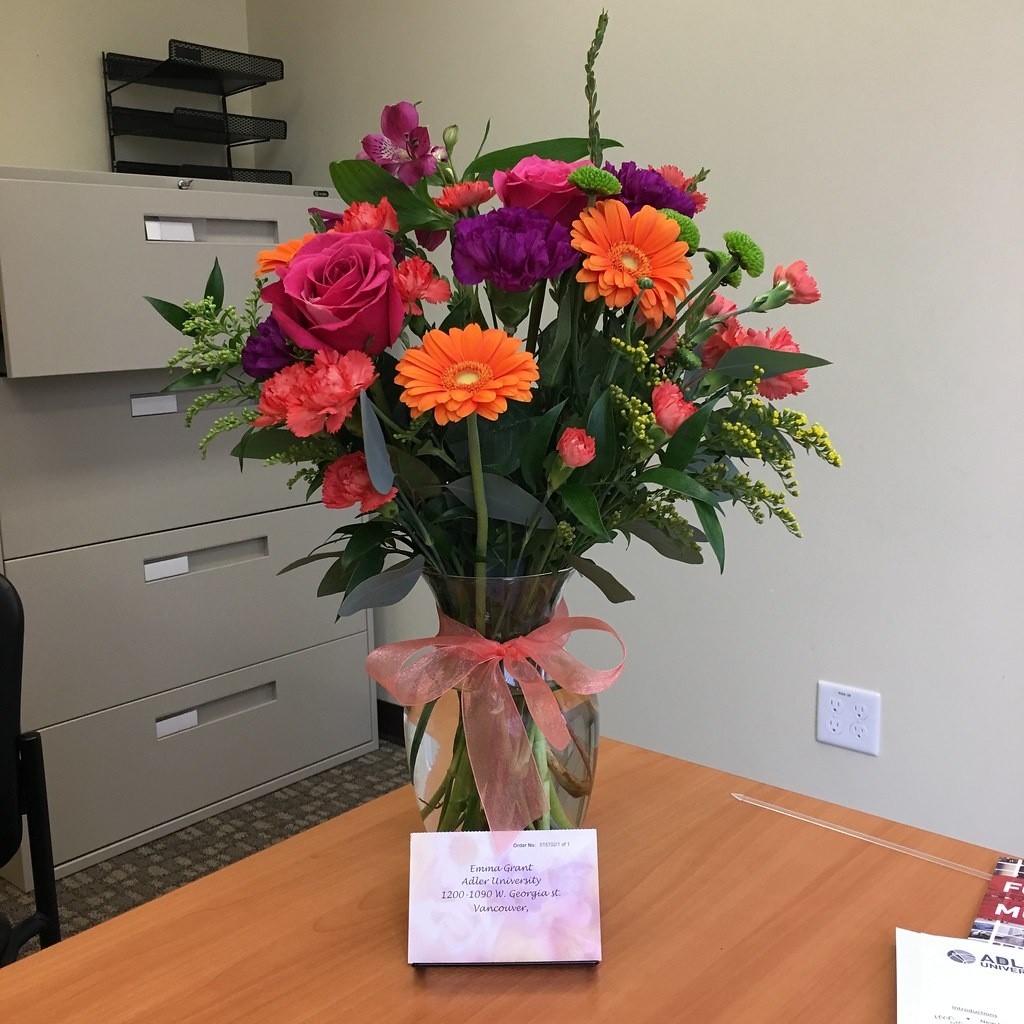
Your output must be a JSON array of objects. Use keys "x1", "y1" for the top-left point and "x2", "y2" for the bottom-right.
[{"x1": 968, "y1": 857, "x2": 1024, "y2": 950}]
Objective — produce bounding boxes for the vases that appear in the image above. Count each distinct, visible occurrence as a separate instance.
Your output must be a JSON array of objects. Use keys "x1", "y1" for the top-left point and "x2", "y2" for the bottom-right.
[{"x1": 401, "y1": 555, "x2": 599, "y2": 835}]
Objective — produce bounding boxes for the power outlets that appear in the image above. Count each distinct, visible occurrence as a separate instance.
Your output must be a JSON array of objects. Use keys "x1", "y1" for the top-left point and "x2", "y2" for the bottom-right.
[{"x1": 816, "y1": 680, "x2": 880, "y2": 756}]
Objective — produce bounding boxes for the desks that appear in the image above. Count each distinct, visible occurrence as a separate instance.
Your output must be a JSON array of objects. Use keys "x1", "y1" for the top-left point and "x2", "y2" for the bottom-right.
[{"x1": 0, "y1": 733, "x2": 1024, "y2": 1024}]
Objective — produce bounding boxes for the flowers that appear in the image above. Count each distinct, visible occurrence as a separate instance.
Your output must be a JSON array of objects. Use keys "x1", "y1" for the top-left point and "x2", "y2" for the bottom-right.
[{"x1": 143, "y1": 11, "x2": 841, "y2": 829}]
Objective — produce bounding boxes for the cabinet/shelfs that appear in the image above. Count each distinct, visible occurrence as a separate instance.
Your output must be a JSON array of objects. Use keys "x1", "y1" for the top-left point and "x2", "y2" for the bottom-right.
[
  {"x1": 0, "y1": 183, "x2": 379, "y2": 887},
  {"x1": 100, "y1": 38, "x2": 291, "y2": 182}
]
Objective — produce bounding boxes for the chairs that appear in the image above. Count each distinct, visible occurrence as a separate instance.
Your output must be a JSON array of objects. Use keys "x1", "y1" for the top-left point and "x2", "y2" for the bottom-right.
[{"x1": 0, "y1": 574, "x2": 61, "y2": 969}]
